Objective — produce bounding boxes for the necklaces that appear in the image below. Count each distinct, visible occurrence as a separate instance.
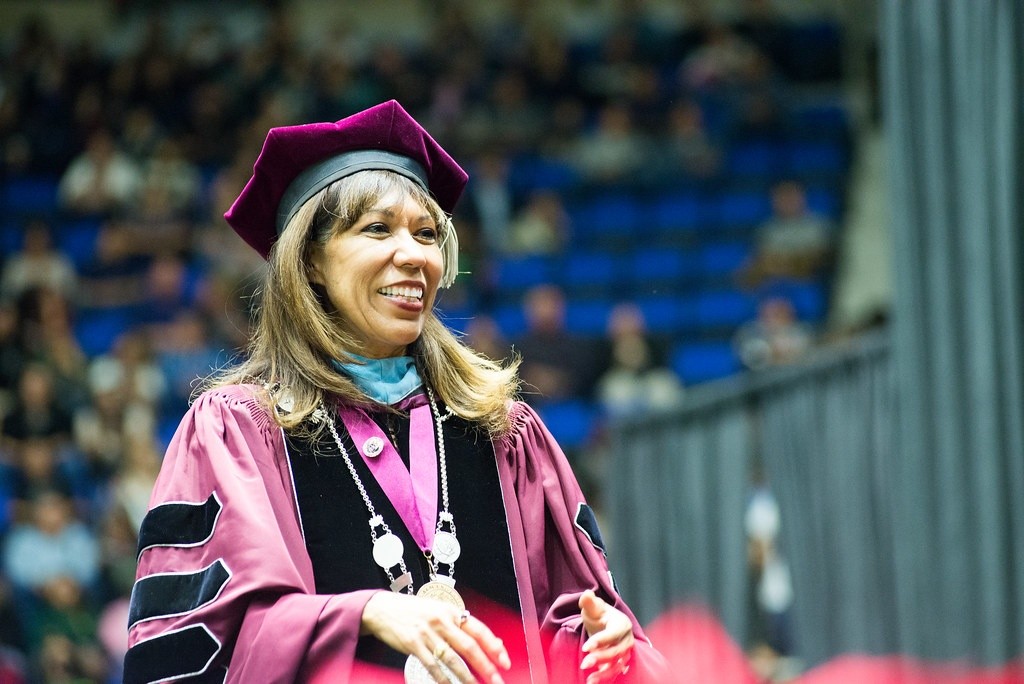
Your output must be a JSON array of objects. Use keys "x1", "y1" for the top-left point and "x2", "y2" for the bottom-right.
[{"x1": 320, "y1": 355, "x2": 466, "y2": 612}]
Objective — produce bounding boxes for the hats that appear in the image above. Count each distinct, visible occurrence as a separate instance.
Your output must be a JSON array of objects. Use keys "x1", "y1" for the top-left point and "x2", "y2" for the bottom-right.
[{"x1": 224, "y1": 98, "x2": 469, "y2": 263}]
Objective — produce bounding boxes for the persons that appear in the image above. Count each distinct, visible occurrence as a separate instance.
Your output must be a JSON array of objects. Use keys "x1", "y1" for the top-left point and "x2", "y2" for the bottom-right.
[
  {"x1": 124, "y1": 101, "x2": 672, "y2": 684},
  {"x1": 0, "y1": 1, "x2": 837, "y2": 684}
]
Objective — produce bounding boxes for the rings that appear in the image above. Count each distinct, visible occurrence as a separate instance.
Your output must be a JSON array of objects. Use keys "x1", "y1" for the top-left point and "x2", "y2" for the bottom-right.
[
  {"x1": 434, "y1": 642, "x2": 450, "y2": 659},
  {"x1": 456, "y1": 611, "x2": 470, "y2": 629}
]
